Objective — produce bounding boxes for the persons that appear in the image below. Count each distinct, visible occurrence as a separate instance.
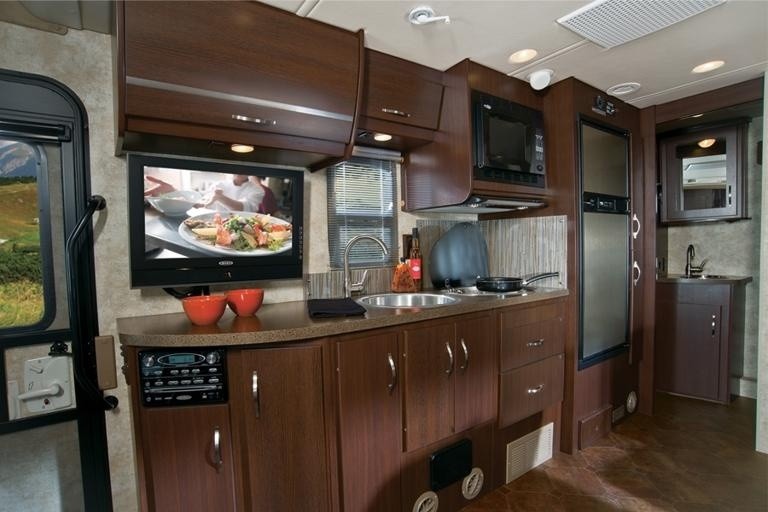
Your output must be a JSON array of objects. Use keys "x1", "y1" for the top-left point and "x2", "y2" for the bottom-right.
[
  {"x1": 249, "y1": 173, "x2": 276, "y2": 214},
  {"x1": 143, "y1": 173, "x2": 266, "y2": 212}
]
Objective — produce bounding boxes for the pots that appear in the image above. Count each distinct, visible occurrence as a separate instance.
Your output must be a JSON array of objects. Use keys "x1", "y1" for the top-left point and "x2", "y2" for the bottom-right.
[{"x1": 475, "y1": 271, "x2": 559, "y2": 293}]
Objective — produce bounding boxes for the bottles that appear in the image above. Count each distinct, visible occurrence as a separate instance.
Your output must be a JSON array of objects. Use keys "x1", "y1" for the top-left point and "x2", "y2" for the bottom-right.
[{"x1": 408, "y1": 226, "x2": 422, "y2": 293}]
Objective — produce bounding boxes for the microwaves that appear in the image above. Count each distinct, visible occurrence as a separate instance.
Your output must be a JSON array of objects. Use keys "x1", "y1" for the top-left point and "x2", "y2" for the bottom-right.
[{"x1": 470, "y1": 88, "x2": 547, "y2": 190}]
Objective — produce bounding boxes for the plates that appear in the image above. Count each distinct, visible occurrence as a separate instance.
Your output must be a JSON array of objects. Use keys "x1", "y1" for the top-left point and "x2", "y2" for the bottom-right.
[{"x1": 176, "y1": 210, "x2": 293, "y2": 258}]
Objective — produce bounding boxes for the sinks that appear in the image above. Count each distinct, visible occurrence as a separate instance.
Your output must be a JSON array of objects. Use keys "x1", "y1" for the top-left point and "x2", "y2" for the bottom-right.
[
  {"x1": 706, "y1": 275, "x2": 726, "y2": 279},
  {"x1": 356, "y1": 292, "x2": 462, "y2": 309},
  {"x1": 681, "y1": 275, "x2": 702, "y2": 279}
]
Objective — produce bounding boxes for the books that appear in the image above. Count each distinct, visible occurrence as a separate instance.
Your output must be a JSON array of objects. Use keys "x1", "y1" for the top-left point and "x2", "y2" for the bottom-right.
[{"x1": 307, "y1": 298, "x2": 367, "y2": 318}]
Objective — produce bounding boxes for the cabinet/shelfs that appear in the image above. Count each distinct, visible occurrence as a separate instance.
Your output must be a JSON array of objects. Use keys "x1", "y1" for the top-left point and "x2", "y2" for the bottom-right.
[
  {"x1": 113, "y1": 1, "x2": 364, "y2": 172},
  {"x1": 357, "y1": 47, "x2": 444, "y2": 146},
  {"x1": 131, "y1": 340, "x2": 339, "y2": 509},
  {"x1": 499, "y1": 294, "x2": 566, "y2": 485},
  {"x1": 398, "y1": 315, "x2": 501, "y2": 511},
  {"x1": 336, "y1": 333, "x2": 403, "y2": 511},
  {"x1": 657, "y1": 281, "x2": 748, "y2": 405}
]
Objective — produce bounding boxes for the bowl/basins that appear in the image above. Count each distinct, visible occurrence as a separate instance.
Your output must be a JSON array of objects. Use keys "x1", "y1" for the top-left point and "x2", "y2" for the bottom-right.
[
  {"x1": 222, "y1": 287, "x2": 264, "y2": 319},
  {"x1": 143, "y1": 189, "x2": 201, "y2": 217},
  {"x1": 180, "y1": 295, "x2": 228, "y2": 327}
]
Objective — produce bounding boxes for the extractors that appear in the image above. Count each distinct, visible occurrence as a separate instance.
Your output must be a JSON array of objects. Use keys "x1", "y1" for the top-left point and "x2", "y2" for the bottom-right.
[{"x1": 408, "y1": 192, "x2": 549, "y2": 214}]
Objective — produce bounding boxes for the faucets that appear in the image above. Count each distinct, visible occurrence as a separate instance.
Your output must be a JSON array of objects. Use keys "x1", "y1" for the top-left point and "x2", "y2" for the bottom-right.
[
  {"x1": 344, "y1": 235, "x2": 389, "y2": 297},
  {"x1": 685, "y1": 245, "x2": 706, "y2": 275}
]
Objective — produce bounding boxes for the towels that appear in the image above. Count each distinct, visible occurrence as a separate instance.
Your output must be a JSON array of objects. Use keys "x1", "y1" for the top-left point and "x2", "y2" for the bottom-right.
[{"x1": 308, "y1": 299, "x2": 367, "y2": 321}]
